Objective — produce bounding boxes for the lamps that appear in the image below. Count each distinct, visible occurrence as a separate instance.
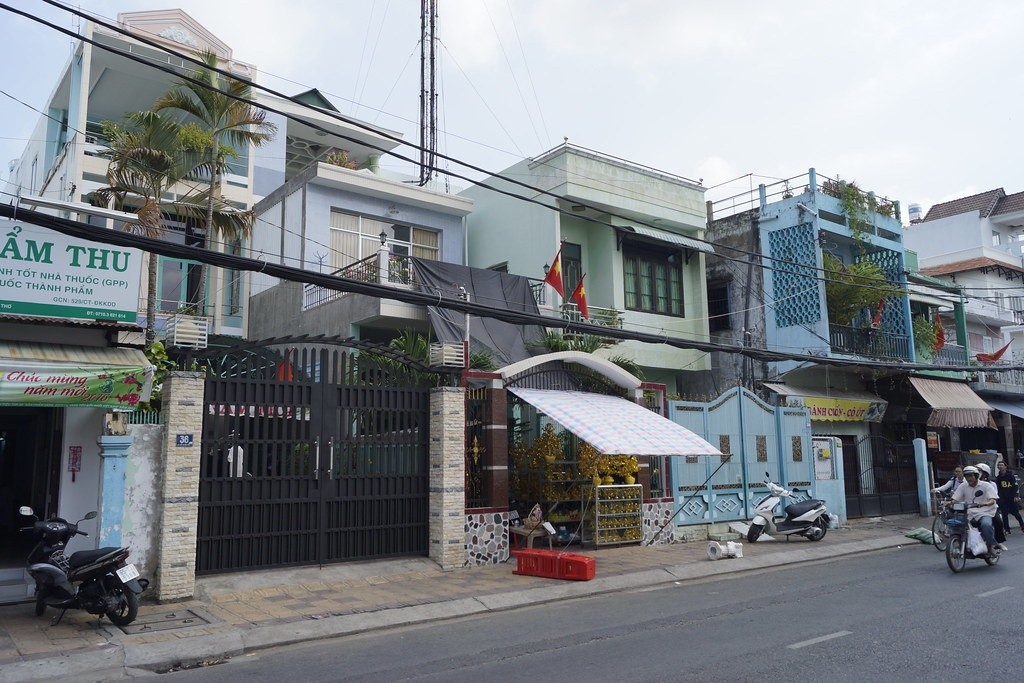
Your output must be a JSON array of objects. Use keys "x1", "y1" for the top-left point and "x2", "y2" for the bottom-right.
[
  {"x1": 543, "y1": 261, "x2": 550, "y2": 274},
  {"x1": 429, "y1": 341, "x2": 463, "y2": 366},
  {"x1": 164, "y1": 314, "x2": 208, "y2": 349},
  {"x1": 379, "y1": 229, "x2": 388, "y2": 246}
]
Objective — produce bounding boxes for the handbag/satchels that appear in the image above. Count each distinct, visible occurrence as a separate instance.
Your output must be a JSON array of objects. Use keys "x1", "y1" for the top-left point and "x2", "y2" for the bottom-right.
[{"x1": 966, "y1": 523, "x2": 988, "y2": 555}]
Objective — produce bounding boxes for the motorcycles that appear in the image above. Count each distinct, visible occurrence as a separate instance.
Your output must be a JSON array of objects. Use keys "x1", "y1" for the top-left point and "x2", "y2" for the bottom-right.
[
  {"x1": 17, "y1": 504, "x2": 149, "y2": 626},
  {"x1": 945, "y1": 490, "x2": 1002, "y2": 574}
]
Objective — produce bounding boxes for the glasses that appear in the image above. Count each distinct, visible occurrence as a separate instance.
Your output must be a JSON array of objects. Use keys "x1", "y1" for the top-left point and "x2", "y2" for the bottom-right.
[{"x1": 954, "y1": 471, "x2": 961, "y2": 473}]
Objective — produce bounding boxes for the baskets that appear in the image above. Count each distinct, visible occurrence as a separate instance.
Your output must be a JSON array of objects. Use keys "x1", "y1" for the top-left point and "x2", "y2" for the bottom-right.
[
  {"x1": 941, "y1": 513, "x2": 968, "y2": 525},
  {"x1": 523, "y1": 504, "x2": 544, "y2": 529}
]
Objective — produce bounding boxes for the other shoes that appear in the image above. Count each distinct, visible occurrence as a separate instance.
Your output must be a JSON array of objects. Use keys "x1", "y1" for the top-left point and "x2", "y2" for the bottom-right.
[
  {"x1": 989, "y1": 544, "x2": 995, "y2": 554},
  {"x1": 1003, "y1": 531, "x2": 1011, "y2": 535},
  {"x1": 1021, "y1": 530, "x2": 1024, "y2": 534},
  {"x1": 999, "y1": 543, "x2": 1008, "y2": 551}
]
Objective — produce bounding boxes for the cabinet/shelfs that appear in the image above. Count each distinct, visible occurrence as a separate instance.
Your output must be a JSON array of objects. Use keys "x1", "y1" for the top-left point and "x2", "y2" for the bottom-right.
[
  {"x1": 538, "y1": 459, "x2": 593, "y2": 546},
  {"x1": 526, "y1": 466, "x2": 540, "y2": 517},
  {"x1": 582, "y1": 484, "x2": 643, "y2": 551}
]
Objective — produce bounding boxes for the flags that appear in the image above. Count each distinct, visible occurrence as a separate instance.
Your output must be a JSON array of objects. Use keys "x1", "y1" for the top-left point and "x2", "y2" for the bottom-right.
[
  {"x1": 873, "y1": 302, "x2": 884, "y2": 325},
  {"x1": 931, "y1": 314, "x2": 944, "y2": 350},
  {"x1": 545, "y1": 255, "x2": 565, "y2": 299},
  {"x1": 976, "y1": 345, "x2": 1008, "y2": 364},
  {"x1": 572, "y1": 280, "x2": 588, "y2": 320}
]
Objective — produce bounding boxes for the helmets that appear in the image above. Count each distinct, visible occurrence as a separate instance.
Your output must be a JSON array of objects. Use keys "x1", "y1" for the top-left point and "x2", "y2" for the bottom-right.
[
  {"x1": 975, "y1": 463, "x2": 991, "y2": 476},
  {"x1": 963, "y1": 466, "x2": 980, "y2": 480}
]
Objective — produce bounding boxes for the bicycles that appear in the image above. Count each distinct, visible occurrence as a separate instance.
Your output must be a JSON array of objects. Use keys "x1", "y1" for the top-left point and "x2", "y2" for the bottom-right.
[{"x1": 932, "y1": 490, "x2": 955, "y2": 551}]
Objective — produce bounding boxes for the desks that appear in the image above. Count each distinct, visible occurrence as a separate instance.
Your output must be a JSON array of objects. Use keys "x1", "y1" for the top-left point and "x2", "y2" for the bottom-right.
[{"x1": 510, "y1": 526, "x2": 553, "y2": 550}]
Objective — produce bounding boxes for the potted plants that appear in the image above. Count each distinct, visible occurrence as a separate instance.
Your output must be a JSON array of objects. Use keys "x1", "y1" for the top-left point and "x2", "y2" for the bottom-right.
[{"x1": 595, "y1": 306, "x2": 622, "y2": 344}]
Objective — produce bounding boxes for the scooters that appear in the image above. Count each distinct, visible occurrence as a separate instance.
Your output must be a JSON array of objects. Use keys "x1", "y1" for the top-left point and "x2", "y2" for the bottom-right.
[{"x1": 747, "y1": 470, "x2": 835, "y2": 543}]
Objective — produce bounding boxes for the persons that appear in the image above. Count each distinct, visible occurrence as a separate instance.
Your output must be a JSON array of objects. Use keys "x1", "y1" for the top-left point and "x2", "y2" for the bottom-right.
[
  {"x1": 996, "y1": 461, "x2": 1024, "y2": 535},
  {"x1": 944, "y1": 466, "x2": 1000, "y2": 553},
  {"x1": 974, "y1": 463, "x2": 1008, "y2": 551},
  {"x1": 932, "y1": 464, "x2": 966, "y2": 493}
]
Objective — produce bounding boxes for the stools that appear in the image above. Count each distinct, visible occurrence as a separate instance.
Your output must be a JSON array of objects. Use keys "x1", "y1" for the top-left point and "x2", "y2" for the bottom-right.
[{"x1": 512, "y1": 548, "x2": 595, "y2": 581}]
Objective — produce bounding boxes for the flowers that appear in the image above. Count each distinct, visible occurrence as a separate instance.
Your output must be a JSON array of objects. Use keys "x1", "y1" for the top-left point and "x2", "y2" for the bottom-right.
[
  {"x1": 327, "y1": 149, "x2": 357, "y2": 170},
  {"x1": 345, "y1": 254, "x2": 409, "y2": 283}
]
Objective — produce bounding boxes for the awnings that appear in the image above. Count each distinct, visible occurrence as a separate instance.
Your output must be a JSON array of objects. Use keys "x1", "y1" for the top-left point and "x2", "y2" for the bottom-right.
[
  {"x1": 504, "y1": 387, "x2": 733, "y2": 563},
  {"x1": 987, "y1": 402, "x2": 1024, "y2": 419},
  {"x1": 761, "y1": 382, "x2": 888, "y2": 423},
  {"x1": 908, "y1": 377, "x2": 995, "y2": 428},
  {"x1": 0, "y1": 342, "x2": 157, "y2": 412},
  {"x1": 618, "y1": 224, "x2": 715, "y2": 264}
]
